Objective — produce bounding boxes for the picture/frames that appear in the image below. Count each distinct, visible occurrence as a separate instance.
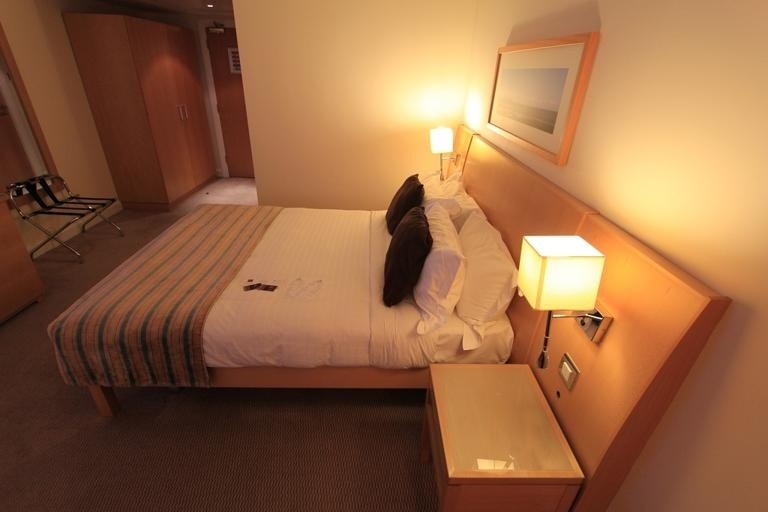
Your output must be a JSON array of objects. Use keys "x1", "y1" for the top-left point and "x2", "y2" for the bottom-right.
[{"x1": 484, "y1": 31, "x2": 601, "y2": 166}]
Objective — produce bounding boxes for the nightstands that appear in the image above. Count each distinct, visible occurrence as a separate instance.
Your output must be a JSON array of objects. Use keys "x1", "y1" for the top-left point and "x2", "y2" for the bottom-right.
[{"x1": 420, "y1": 363, "x2": 586, "y2": 512}]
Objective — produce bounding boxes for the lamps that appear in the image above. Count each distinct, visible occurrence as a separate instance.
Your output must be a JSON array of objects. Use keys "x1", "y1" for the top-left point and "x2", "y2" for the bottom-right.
[
  {"x1": 517, "y1": 235, "x2": 606, "y2": 369},
  {"x1": 430, "y1": 127, "x2": 459, "y2": 181}
]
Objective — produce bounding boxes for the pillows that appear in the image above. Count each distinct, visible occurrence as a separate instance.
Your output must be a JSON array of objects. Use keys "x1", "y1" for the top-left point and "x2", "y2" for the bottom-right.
[{"x1": 382, "y1": 167, "x2": 524, "y2": 351}]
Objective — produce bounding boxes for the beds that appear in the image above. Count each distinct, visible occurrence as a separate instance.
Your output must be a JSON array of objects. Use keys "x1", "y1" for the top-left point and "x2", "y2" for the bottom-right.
[{"x1": 46, "y1": 204, "x2": 515, "y2": 417}]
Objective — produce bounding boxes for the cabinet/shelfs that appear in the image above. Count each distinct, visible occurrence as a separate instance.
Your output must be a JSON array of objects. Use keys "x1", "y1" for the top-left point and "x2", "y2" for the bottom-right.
[
  {"x1": 8, "y1": 174, "x2": 125, "y2": 264},
  {"x1": 62, "y1": 12, "x2": 217, "y2": 212}
]
